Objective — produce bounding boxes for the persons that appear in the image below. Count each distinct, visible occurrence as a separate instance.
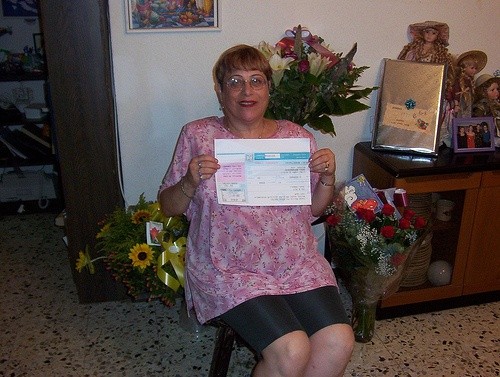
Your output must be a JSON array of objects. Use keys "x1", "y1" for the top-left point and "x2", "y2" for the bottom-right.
[
  {"x1": 455, "y1": 49, "x2": 482, "y2": 119},
  {"x1": 472, "y1": 74, "x2": 500, "y2": 148},
  {"x1": 157, "y1": 44, "x2": 359, "y2": 377},
  {"x1": 395, "y1": 20, "x2": 458, "y2": 150},
  {"x1": 458, "y1": 122, "x2": 491, "y2": 149}
]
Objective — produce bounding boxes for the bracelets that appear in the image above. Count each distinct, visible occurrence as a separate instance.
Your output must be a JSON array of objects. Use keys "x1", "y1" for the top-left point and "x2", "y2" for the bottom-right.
[
  {"x1": 180, "y1": 176, "x2": 194, "y2": 199},
  {"x1": 319, "y1": 179, "x2": 334, "y2": 186}
]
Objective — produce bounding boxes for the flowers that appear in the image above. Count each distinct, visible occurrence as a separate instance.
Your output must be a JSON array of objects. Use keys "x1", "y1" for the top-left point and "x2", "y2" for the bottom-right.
[
  {"x1": 252, "y1": 25, "x2": 382, "y2": 137},
  {"x1": 75, "y1": 192, "x2": 189, "y2": 308},
  {"x1": 321, "y1": 199, "x2": 427, "y2": 341}
]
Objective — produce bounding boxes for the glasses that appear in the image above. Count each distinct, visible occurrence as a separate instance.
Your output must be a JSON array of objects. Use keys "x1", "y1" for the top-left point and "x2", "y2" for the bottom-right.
[{"x1": 220, "y1": 75, "x2": 269, "y2": 91}]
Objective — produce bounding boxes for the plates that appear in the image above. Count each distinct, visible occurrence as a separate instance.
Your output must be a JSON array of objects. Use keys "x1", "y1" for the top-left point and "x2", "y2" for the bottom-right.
[
  {"x1": 400, "y1": 232, "x2": 433, "y2": 287},
  {"x1": 406, "y1": 192, "x2": 432, "y2": 226}
]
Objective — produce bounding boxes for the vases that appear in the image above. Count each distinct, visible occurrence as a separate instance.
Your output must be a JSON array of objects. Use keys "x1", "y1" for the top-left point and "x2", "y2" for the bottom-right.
[{"x1": 355, "y1": 297, "x2": 377, "y2": 342}]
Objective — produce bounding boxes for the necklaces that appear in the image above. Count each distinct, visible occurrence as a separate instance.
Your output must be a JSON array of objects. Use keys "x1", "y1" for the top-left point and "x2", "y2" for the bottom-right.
[{"x1": 222, "y1": 121, "x2": 265, "y2": 139}]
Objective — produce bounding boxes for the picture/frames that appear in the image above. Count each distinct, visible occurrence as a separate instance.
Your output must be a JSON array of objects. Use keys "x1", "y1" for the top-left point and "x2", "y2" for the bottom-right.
[
  {"x1": 124, "y1": 0, "x2": 222, "y2": 34},
  {"x1": 452, "y1": 117, "x2": 495, "y2": 153}
]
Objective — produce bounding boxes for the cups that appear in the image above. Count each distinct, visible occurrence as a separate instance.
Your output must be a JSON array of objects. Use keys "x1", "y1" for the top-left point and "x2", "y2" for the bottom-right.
[{"x1": 436, "y1": 199, "x2": 455, "y2": 221}]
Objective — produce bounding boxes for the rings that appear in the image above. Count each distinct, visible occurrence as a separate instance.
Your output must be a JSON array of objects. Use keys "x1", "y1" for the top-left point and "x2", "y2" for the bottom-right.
[
  {"x1": 200, "y1": 176, "x2": 203, "y2": 180},
  {"x1": 198, "y1": 168, "x2": 202, "y2": 176},
  {"x1": 198, "y1": 161, "x2": 202, "y2": 168},
  {"x1": 325, "y1": 162, "x2": 329, "y2": 171}
]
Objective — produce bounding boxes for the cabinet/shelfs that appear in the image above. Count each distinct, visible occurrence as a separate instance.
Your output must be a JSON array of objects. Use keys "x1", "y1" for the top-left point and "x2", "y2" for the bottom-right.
[
  {"x1": 0, "y1": 64, "x2": 56, "y2": 168},
  {"x1": 352, "y1": 144, "x2": 500, "y2": 310}
]
